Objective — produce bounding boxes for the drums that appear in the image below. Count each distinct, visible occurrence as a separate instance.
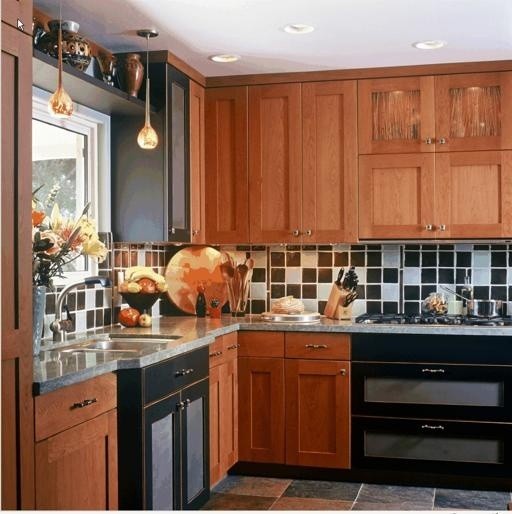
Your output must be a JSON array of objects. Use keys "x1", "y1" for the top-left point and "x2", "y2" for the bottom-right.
[{"x1": 336, "y1": 265, "x2": 359, "y2": 292}]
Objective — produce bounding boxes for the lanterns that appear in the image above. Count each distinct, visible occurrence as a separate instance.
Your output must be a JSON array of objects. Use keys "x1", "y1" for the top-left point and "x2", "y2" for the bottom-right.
[{"x1": 127, "y1": 282, "x2": 142, "y2": 292}]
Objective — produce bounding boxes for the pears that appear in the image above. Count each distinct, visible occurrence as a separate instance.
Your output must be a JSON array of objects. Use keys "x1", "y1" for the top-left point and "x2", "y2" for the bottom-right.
[{"x1": 460, "y1": 276, "x2": 473, "y2": 315}]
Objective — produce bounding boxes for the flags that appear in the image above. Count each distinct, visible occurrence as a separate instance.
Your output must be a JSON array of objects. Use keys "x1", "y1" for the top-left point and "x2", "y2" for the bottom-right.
[
  {"x1": 33, "y1": 284, "x2": 48, "y2": 356},
  {"x1": 116, "y1": 53, "x2": 144, "y2": 98}
]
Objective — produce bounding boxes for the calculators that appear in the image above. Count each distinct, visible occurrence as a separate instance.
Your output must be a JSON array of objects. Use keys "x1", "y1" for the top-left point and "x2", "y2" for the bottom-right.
[
  {"x1": 119, "y1": 308, "x2": 140, "y2": 327},
  {"x1": 137, "y1": 278, "x2": 156, "y2": 295}
]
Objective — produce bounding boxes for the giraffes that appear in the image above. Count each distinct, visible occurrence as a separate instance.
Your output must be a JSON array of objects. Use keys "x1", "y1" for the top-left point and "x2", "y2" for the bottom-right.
[{"x1": 354, "y1": 310, "x2": 511, "y2": 326}]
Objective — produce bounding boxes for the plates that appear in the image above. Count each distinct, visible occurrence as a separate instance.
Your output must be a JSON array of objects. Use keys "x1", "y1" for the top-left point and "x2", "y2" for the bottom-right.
[
  {"x1": 2, "y1": 0, "x2": 33, "y2": 511},
  {"x1": 188, "y1": 75, "x2": 203, "y2": 246},
  {"x1": 236, "y1": 326, "x2": 285, "y2": 466},
  {"x1": 250, "y1": 78, "x2": 357, "y2": 246},
  {"x1": 347, "y1": 331, "x2": 512, "y2": 493},
  {"x1": 114, "y1": 63, "x2": 192, "y2": 247},
  {"x1": 207, "y1": 329, "x2": 237, "y2": 484},
  {"x1": 285, "y1": 329, "x2": 352, "y2": 472},
  {"x1": 358, "y1": 69, "x2": 512, "y2": 157},
  {"x1": 356, "y1": 149, "x2": 512, "y2": 241},
  {"x1": 117, "y1": 344, "x2": 209, "y2": 509},
  {"x1": 203, "y1": 86, "x2": 249, "y2": 245},
  {"x1": 32, "y1": 371, "x2": 118, "y2": 509}
]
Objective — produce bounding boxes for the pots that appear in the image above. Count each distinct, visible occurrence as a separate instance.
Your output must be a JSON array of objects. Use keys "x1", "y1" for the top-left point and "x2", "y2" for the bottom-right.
[{"x1": 445, "y1": 299, "x2": 506, "y2": 318}]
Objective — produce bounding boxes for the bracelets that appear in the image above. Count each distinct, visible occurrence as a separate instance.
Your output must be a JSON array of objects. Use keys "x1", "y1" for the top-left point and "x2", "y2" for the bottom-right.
[{"x1": 119, "y1": 287, "x2": 162, "y2": 309}]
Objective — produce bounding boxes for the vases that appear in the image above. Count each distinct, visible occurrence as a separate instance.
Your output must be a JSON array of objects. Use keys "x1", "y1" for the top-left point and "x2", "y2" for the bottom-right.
[{"x1": 31, "y1": 178, "x2": 111, "y2": 287}]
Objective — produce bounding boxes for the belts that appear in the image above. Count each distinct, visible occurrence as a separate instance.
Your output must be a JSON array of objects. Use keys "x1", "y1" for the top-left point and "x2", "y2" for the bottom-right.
[{"x1": 96, "y1": 53, "x2": 117, "y2": 86}]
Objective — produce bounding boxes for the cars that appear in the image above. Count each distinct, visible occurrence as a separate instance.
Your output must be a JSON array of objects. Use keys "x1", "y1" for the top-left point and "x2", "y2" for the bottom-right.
[{"x1": 222, "y1": 268, "x2": 253, "y2": 316}]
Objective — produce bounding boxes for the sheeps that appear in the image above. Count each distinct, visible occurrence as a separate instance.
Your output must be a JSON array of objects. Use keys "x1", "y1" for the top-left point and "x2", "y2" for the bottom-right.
[{"x1": 50, "y1": 275, "x2": 114, "y2": 343}]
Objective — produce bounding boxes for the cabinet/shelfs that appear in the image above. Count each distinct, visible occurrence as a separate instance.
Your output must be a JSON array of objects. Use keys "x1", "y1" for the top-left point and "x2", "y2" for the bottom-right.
[
  {"x1": 196, "y1": 286, "x2": 207, "y2": 317},
  {"x1": 196, "y1": 316, "x2": 207, "y2": 337}
]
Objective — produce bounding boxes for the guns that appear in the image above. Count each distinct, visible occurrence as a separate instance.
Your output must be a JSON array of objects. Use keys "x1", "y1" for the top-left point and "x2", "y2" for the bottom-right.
[{"x1": 125, "y1": 266, "x2": 166, "y2": 288}]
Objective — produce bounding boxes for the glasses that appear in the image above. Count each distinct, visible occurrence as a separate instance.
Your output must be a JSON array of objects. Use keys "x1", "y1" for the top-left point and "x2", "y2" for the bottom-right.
[
  {"x1": 46, "y1": 0, "x2": 75, "y2": 122},
  {"x1": 134, "y1": 27, "x2": 161, "y2": 153}
]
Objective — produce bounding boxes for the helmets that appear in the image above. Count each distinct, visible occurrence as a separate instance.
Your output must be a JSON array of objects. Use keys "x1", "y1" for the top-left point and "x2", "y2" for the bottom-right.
[{"x1": 165, "y1": 246, "x2": 232, "y2": 314}]
